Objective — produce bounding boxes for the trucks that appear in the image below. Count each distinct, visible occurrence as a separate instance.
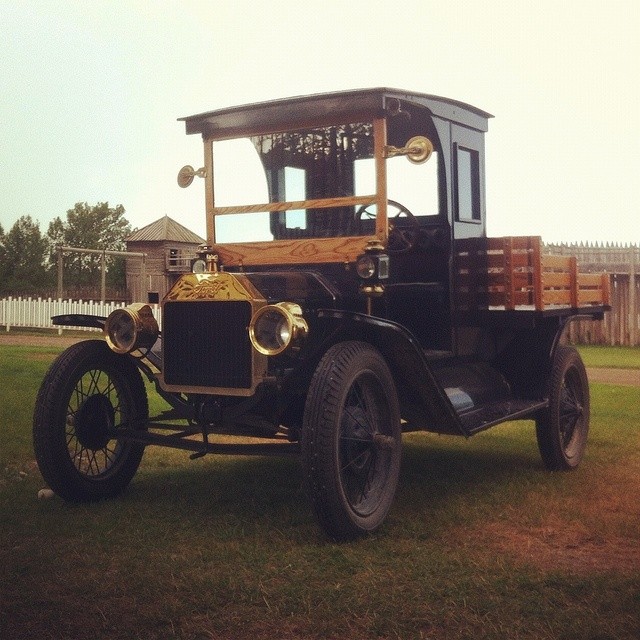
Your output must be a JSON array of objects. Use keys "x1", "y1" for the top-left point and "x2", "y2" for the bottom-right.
[{"x1": 33, "y1": 89, "x2": 613, "y2": 541}]
